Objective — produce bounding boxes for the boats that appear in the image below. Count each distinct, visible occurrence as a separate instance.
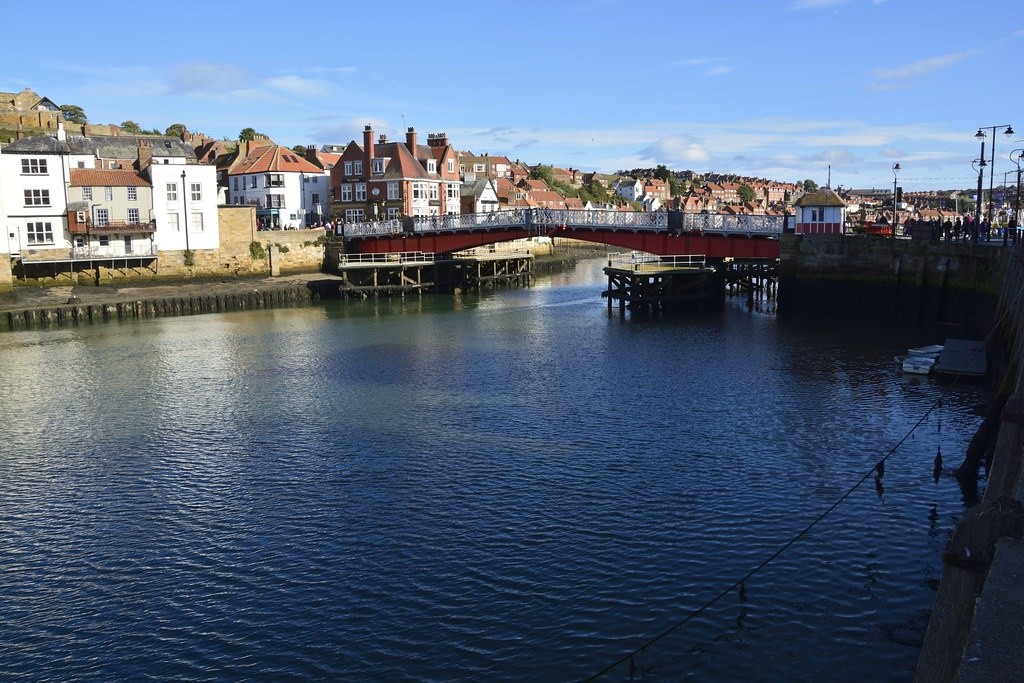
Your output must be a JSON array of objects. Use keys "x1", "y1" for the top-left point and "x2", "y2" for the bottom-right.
[{"x1": 902, "y1": 345, "x2": 944, "y2": 374}]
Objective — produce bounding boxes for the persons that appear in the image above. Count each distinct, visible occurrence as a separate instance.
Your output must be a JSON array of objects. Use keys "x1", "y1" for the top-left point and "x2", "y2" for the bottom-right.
[
  {"x1": 875, "y1": 213, "x2": 887, "y2": 224},
  {"x1": 262, "y1": 219, "x2": 378, "y2": 234},
  {"x1": 432, "y1": 213, "x2": 448, "y2": 228},
  {"x1": 487, "y1": 206, "x2": 568, "y2": 225},
  {"x1": 1008, "y1": 217, "x2": 1015, "y2": 236},
  {"x1": 904, "y1": 216, "x2": 988, "y2": 241}
]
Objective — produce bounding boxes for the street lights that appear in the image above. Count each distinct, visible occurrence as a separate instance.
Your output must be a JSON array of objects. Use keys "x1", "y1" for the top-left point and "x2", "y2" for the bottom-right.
[
  {"x1": 1010, "y1": 149, "x2": 1024, "y2": 243},
  {"x1": 892, "y1": 161, "x2": 902, "y2": 223},
  {"x1": 974, "y1": 125, "x2": 1015, "y2": 242},
  {"x1": 971, "y1": 142, "x2": 992, "y2": 238},
  {"x1": 180, "y1": 170, "x2": 189, "y2": 251}
]
